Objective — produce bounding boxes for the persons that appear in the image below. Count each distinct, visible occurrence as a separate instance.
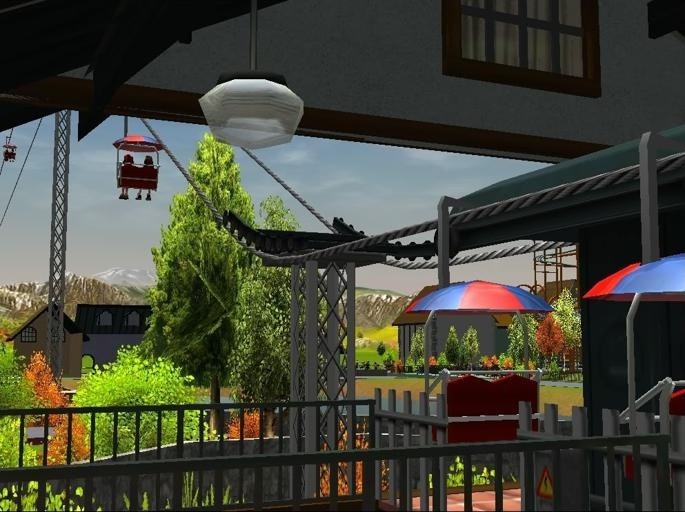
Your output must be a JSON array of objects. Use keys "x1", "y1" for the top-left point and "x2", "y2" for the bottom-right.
[
  {"x1": 4, "y1": 150, "x2": 9, "y2": 161},
  {"x1": 9, "y1": 149, "x2": 15, "y2": 162},
  {"x1": 117, "y1": 155, "x2": 132, "y2": 200},
  {"x1": 137, "y1": 156, "x2": 154, "y2": 200}
]
